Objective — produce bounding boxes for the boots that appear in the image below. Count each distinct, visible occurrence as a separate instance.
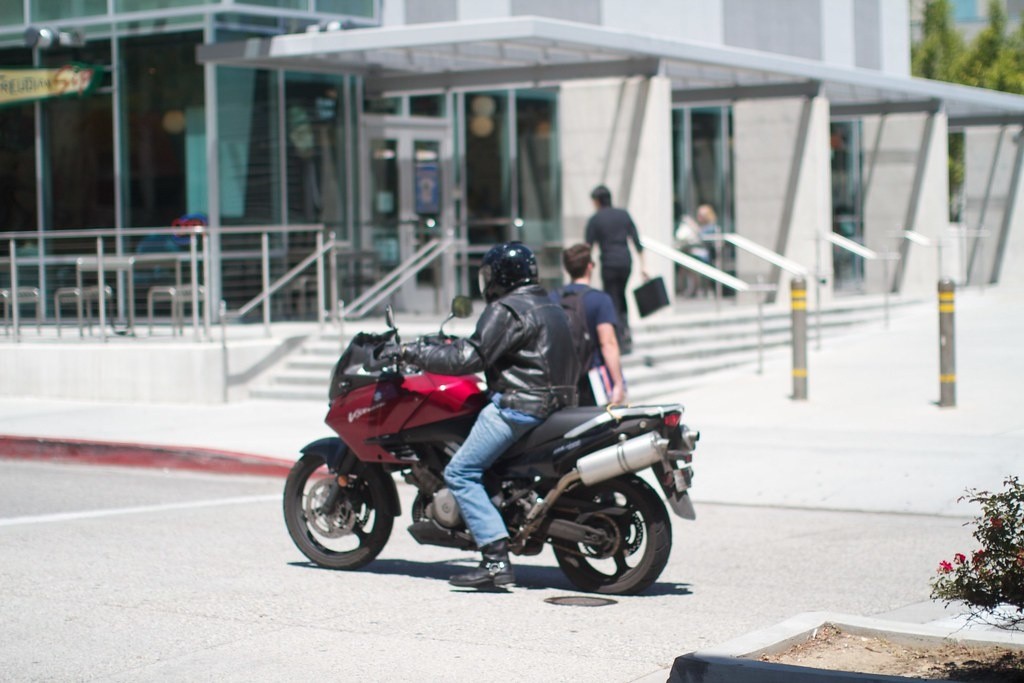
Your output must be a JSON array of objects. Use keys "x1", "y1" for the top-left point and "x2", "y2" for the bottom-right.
[{"x1": 448, "y1": 540, "x2": 515, "y2": 587}]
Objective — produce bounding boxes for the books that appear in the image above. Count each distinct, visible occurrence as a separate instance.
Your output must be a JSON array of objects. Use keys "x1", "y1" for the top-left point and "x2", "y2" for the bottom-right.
[{"x1": 588, "y1": 364, "x2": 629, "y2": 409}]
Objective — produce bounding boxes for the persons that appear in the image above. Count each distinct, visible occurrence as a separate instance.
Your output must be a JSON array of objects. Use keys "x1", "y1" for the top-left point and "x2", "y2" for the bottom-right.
[
  {"x1": 583, "y1": 185, "x2": 647, "y2": 354},
  {"x1": 677, "y1": 204, "x2": 720, "y2": 298},
  {"x1": 545, "y1": 243, "x2": 627, "y2": 407},
  {"x1": 379, "y1": 242, "x2": 576, "y2": 585}
]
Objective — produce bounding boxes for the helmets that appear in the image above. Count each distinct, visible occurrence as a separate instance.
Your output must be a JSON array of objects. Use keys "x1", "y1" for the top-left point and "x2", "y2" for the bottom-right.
[{"x1": 476, "y1": 240, "x2": 538, "y2": 303}]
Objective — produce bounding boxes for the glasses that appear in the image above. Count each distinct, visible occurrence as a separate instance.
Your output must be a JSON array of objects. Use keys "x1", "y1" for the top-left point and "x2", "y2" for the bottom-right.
[{"x1": 589, "y1": 260, "x2": 595, "y2": 267}]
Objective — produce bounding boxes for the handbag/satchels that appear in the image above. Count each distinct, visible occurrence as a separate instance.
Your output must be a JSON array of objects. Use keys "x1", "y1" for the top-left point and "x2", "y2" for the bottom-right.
[{"x1": 633, "y1": 276, "x2": 669, "y2": 318}]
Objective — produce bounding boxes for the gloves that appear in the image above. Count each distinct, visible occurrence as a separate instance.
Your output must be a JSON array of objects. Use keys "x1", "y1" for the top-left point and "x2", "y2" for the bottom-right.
[{"x1": 378, "y1": 346, "x2": 402, "y2": 362}]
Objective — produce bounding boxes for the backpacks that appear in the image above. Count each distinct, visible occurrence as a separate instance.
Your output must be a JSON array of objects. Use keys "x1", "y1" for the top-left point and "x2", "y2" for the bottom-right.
[{"x1": 556, "y1": 287, "x2": 594, "y2": 376}]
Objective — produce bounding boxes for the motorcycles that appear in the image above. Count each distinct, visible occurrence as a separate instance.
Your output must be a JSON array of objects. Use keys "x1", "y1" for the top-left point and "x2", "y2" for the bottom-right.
[{"x1": 280, "y1": 294, "x2": 702, "y2": 595}]
